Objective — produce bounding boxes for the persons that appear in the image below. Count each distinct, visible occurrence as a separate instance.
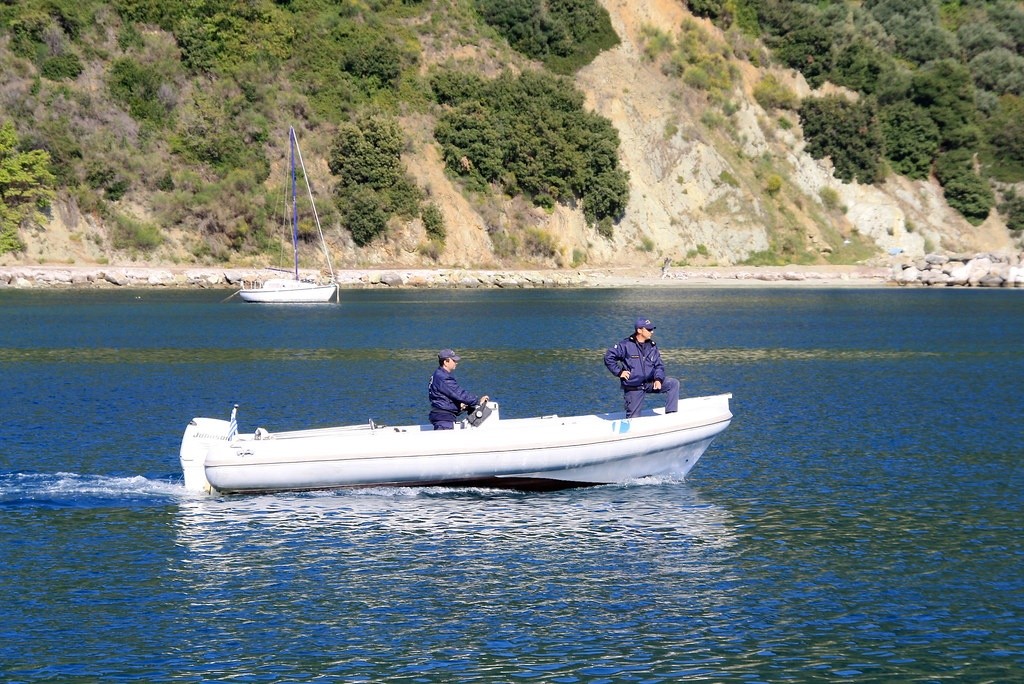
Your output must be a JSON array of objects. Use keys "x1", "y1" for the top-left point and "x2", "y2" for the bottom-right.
[
  {"x1": 605, "y1": 318, "x2": 679, "y2": 419},
  {"x1": 428, "y1": 349, "x2": 489, "y2": 430}
]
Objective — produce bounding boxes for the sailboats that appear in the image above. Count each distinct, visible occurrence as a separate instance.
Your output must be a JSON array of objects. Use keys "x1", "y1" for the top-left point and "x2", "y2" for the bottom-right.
[{"x1": 238, "y1": 124, "x2": 340, "y2": 303}]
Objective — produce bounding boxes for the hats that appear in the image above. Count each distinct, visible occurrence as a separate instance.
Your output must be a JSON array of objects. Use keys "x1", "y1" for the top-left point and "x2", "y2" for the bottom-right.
[
  {"x1": 634, "y1": 318, "x2": 657, "y2": 329},
  {"x1": 438, "y1": 349, "x2": 461, "y2": 360}
]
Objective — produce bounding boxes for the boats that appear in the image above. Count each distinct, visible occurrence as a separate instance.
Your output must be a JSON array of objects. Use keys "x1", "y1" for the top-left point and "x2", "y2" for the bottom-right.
[{"x1": 182, "y1": 393, "x2": 736, "y2": 494}]
